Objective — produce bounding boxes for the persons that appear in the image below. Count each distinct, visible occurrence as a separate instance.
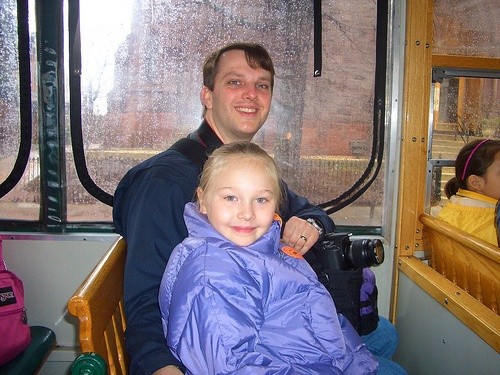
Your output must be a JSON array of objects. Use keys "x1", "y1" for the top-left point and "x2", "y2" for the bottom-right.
[
  {"x1": 431, "y1": 138, "x2": 500, "y2": 247},
  {"x1": 111, "y1": 43, "x2": 411, "y2": 375},
  {"x1": 157, "y1": 140, "x2": 383, "y2": 375}
]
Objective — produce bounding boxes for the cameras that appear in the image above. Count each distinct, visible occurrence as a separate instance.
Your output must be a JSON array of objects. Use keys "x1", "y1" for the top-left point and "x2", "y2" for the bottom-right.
[{"x1": 318, "y1": 232, "x2": 384, "y2": 271}]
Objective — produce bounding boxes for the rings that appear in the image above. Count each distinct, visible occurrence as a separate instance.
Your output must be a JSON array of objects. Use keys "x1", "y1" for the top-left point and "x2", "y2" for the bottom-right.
[{"x1": 300, "y1": 236, "x2": 307, "y2": 241}]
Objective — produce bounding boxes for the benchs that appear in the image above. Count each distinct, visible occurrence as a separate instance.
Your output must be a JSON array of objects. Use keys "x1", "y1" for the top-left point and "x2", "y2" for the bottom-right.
[
  {"x1": 419, "y1": 213, "x2": 500, "y2": 319},
  {"x1": 68, "y1": 235, "x2": 130, "y2": 375}
]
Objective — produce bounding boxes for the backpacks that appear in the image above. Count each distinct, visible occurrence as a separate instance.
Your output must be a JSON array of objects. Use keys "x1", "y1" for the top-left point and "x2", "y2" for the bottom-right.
[{"x1": 0, "y1": 237, "x2": 31, "y2": 365}]
